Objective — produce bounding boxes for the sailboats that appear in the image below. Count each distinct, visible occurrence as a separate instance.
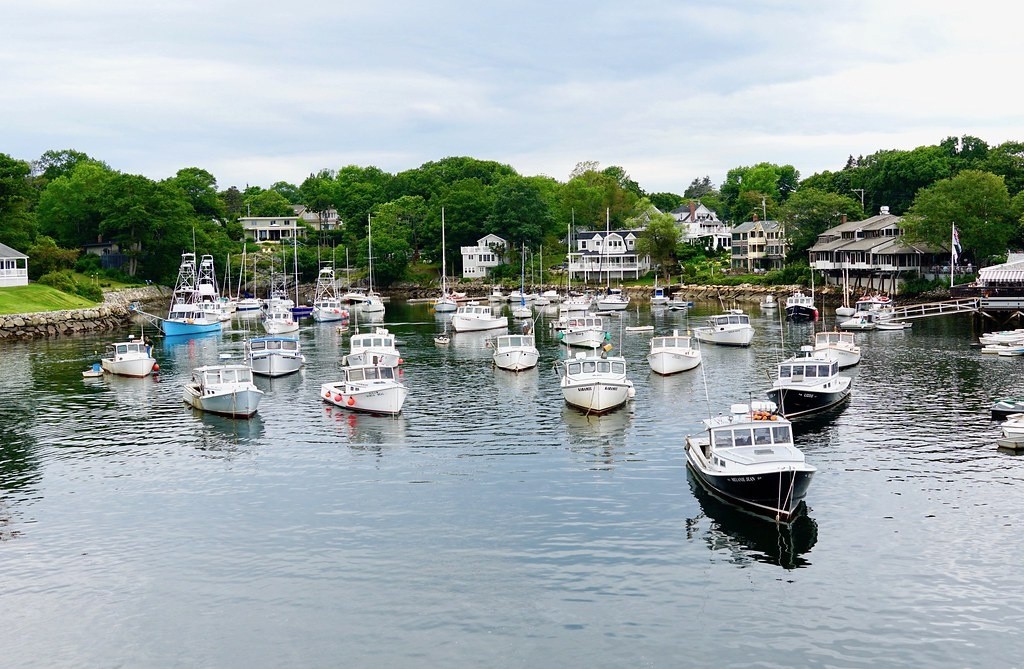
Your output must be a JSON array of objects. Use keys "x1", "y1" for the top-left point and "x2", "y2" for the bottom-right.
[{"x1": 131, "y1": 203, "x2": 690, "y2": 372}]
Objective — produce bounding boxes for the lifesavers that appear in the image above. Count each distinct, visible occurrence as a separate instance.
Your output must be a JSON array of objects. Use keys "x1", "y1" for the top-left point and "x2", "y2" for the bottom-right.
[
  {"x1": 858, "y1": 296, "x2": 870, "y2": 300},
  {"x1": 877, "y1": 296, "x2": 889, "y2": 301}
]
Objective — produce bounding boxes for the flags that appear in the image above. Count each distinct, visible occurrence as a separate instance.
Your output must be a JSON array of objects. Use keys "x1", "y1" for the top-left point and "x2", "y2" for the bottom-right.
[{"x1": 952, "y1": 227, "x2": 962, "y2": 263}]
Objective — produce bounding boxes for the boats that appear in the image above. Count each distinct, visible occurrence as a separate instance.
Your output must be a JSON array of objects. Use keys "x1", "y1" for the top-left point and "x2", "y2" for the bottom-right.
[
  {"x1": 979, "y1": 329, "x2": 1024, "y2": 356},
  {"x1": 760, "y1": 295, "x2": 779, "y2": 310},
  {"x1": 695, "y1": 301, "x2": 756, "y2": 348},
  {"x1": 552, "y1": 311, "x2": 636, "y2": 412},
  {"x1": 182, "y1": 361, "x2": 265, "y2": 420},
  {"x1": 998, "y1": 414, "x2": 1024, "y2": 453},
  {"x1": 319, "y1": 363, "x2": 410, "y2": 420},
  {"x1": 647, "y1": 328, "x2": 702, "y2": 375},
  {"x1": 989, "y1": 396, "x2": 1024, "y2": 417},
  {"x1": 684, "y1": 333, "x2": 819, "y2": 525},
  {"x1": 82, "y1": 364, "x2": 105, "y2": 378},
  {"x1": 102, "y1": 324, "x2": 161, "y2": 378},
  {"x1": 242, "y1": 335, "x2": 305, "y2": 379},
  {"x1": 766, "y1": 262, "x2": 912, "y2": 425}
]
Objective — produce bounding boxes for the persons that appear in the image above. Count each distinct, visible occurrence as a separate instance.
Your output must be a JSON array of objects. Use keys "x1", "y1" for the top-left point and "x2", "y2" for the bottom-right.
[
  {"x1": 144, "y1": 336, "x2": 153, "y2": 358},
  {"x1": 621, "y1": 285, "x2": 628, "y2": 301},
  {"x1": 929, "y1": 260, "x2": 972, "y2": 274}
]
